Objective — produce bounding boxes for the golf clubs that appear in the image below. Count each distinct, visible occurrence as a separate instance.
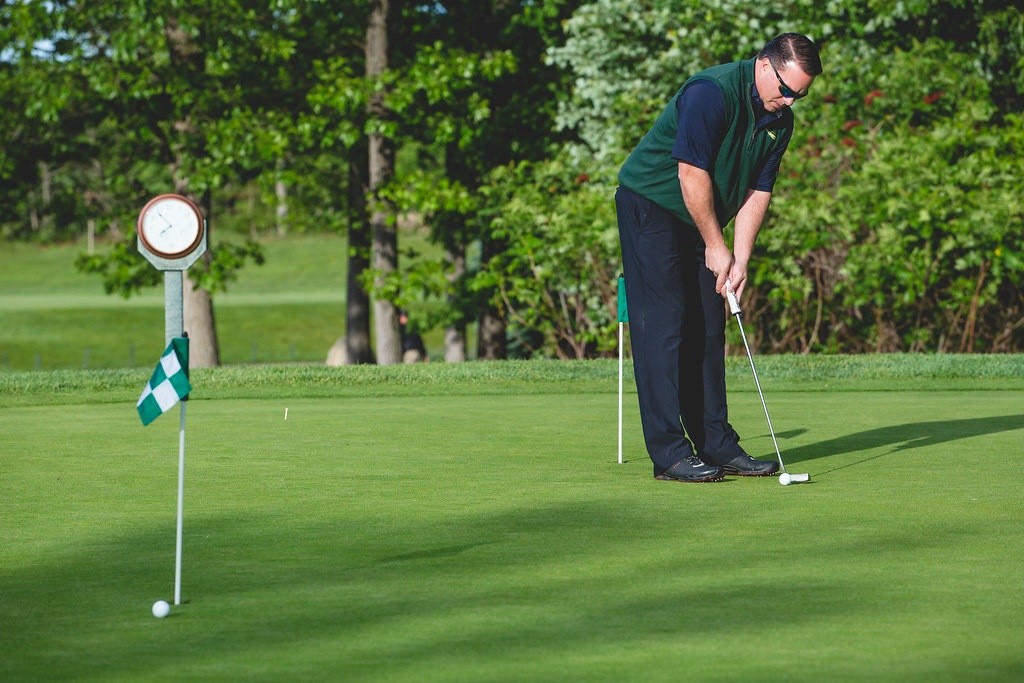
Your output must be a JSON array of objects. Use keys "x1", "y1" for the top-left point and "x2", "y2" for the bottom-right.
[{"x1": 726, "y1": 278, "x2": 812, "y2": 483}]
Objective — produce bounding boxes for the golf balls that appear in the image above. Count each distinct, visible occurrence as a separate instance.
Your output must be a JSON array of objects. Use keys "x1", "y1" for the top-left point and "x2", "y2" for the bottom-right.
[
  {"x1": 152, "y1": 600, "x2": 171, "y2": 618},
  {"x1": 779, "y1": 473, "x2": 792, "y2": 485}
]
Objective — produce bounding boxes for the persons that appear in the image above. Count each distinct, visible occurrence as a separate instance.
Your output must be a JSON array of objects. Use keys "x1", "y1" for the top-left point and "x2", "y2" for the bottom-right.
[{"x1": 615, "y1": 33, "x2": 824, "y2": 484}]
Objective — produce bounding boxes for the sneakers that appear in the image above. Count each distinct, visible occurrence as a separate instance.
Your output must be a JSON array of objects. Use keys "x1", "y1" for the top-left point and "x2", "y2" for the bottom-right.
[
  {"x1": 656, "y1": 454, "x2": 722, "y2": 483},
  {"x1": 721, "y1": 451, "x2": 780, "y2": 477}
]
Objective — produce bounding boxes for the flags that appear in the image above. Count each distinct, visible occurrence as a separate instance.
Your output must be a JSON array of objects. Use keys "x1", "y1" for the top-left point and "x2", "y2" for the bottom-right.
[
  {"x1": 136, "y1": 336, "x2": 192, "y2": 427},
  {"x1": 617, "y1": 275, "x2": 628, "y2": 323}
]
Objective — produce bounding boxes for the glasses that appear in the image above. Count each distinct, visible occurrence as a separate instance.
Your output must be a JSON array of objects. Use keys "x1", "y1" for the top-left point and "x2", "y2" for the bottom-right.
[{"x1": 765, "y1": 55, "x2": 808, "y2": 99}]
